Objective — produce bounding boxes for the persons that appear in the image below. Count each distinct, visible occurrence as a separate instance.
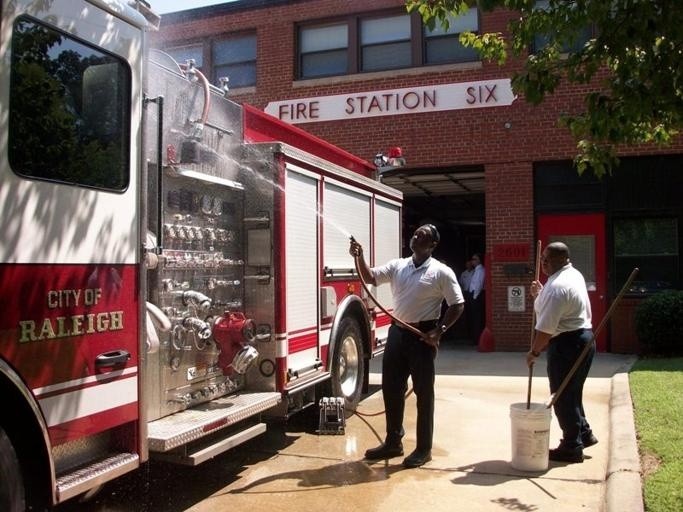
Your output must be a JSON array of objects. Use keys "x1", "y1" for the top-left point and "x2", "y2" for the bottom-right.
[
  {"x1": 459, "y1": 260, "x2": 475, "y2": 335},
  {"x1": 525, "y1": 239, "x2": 599, "y2": 463},
  {"x1": 347, "y1": 222, "x2": 468, "y2": 469},
  {"x1": 468, "y1": 254, "x2": 485, "y2": 348}
]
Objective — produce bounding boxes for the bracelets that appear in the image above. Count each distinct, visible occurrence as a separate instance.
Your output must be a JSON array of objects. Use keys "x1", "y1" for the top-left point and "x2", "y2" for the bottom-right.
[{"x1": 529, "y1": 350, "x2": 540, "y2": 359}]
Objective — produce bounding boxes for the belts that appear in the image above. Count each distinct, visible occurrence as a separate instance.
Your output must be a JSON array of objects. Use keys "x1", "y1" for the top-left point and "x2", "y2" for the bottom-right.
[{"x1": 391, "y1": 317, "x2": 439, "y2": 331}]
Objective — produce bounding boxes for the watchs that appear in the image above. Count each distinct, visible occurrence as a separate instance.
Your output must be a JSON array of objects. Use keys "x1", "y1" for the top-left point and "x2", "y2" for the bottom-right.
[{"x1": 439, "y1": 322, "x2": 447, "y2": 332}]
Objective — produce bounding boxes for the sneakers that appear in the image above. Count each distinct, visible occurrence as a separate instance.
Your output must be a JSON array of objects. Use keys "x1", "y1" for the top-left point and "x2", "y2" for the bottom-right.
[
  {"x1": 547, "y1": 438, "x2": 585, "y2": 464},
  {"x1": 579, "y1": 428, "x2": 600, "y2": 450},
  {"x1": 362, "y1": 434, "x2": 404, "y2": 462},
  {"x1": 400, "y1": 447, "x2": 434, "y2": 469}
]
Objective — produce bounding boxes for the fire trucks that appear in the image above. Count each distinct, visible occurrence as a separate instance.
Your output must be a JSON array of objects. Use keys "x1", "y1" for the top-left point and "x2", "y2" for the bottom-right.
[{"x1": 0, "y1": 0, "x2": 406, "y2": 512}]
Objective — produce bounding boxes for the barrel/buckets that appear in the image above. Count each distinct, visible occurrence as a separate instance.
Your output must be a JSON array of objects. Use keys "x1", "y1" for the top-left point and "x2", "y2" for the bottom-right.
[
  {"x1": 510, "y1": 403, "x2": 553, "y2": 472},
  {"x1": 478, "y1": 326, "x2": 494, "y2": 351}
]
